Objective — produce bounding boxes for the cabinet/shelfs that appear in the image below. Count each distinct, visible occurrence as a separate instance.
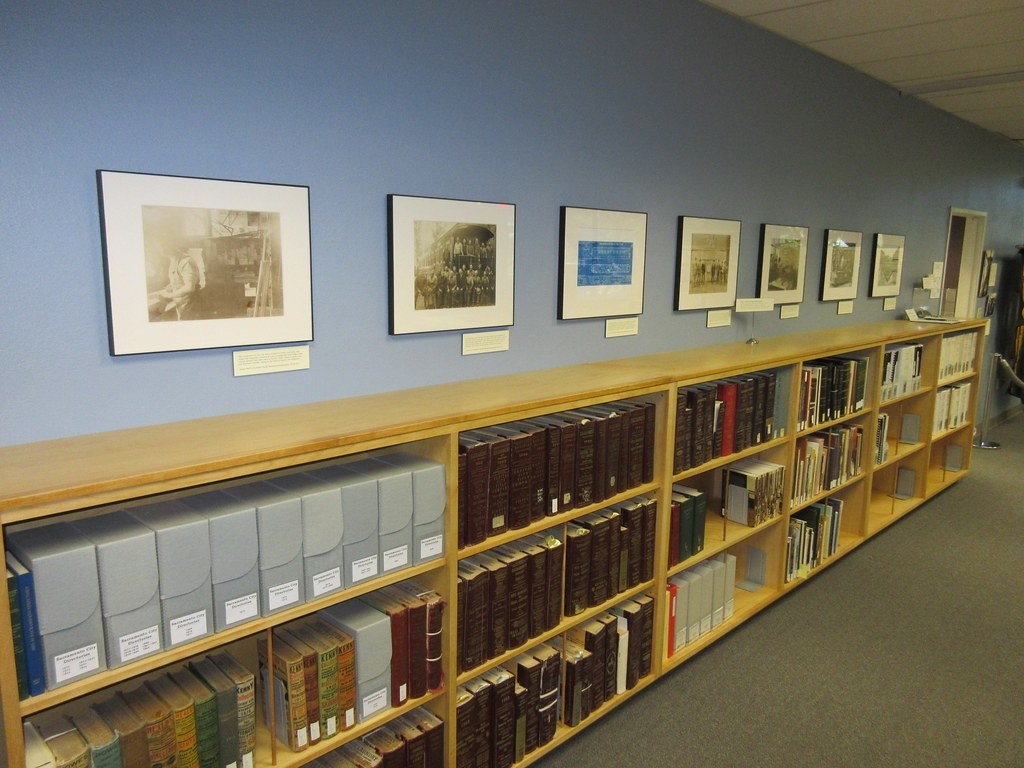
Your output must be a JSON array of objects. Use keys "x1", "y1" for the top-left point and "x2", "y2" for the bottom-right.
[{"x1": 0, "y1": 318, "x2": 990, "y2": 768}]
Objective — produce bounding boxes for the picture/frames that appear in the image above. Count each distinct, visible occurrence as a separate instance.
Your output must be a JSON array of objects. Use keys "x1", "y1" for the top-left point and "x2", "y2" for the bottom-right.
[
  {"x1": 867, "y1": 233, "x2": 906, "y2": 297},
  {"x1": 556, "y1": 205, "x2": 648, "y2": 320},
  {"x1": 817, "y1": 228, "x2": 863, "y2": 301},
  {"x1": 755, "y1": 223, "x2": 809, "y2": 304},
  {"x1": 386, "y1": 194, "x2": 516, "y2": 335},
  {"x1": 95, "y1": 169, "x2": 313, "y2": 356},
  {"x1": 673, "y1": 216, "x2": 741, "y2": 311}
]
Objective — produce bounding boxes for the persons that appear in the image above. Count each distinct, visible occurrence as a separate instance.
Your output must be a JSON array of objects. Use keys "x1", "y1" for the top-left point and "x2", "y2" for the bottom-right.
[
  {"x1": 415, "y1": 237, "x2": 494, "y2": 309},
  {"x1": 148, "y1": 238, "x2": 199, "y2": 321},
  {"x1": 693, "y1": 257, "x2": 728, "y2": 286}
]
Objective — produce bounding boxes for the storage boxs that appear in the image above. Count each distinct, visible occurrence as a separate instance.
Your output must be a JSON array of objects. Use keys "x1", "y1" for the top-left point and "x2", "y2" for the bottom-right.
[
  {"x1": 670, "y1": 553, "x2": 737, "y2": 654},
  {"x1": 321, "y1": 596, "x2": 392, "y2": 727},
  {"x1": 7, "y1": 452, "x2": 448, "y2": 692}
]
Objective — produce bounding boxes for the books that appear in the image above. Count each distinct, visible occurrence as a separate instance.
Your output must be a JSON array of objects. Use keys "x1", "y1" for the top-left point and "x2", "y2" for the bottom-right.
[
  {"x1": 932, "y1": 332, "x2": 978, "y2": 435},
  {"x1": 0, "y1": 356, "x2": 870, "y2": 768},
  {"x1": 874, "y1": 343, "x2": 924, "y2": 467}
]
